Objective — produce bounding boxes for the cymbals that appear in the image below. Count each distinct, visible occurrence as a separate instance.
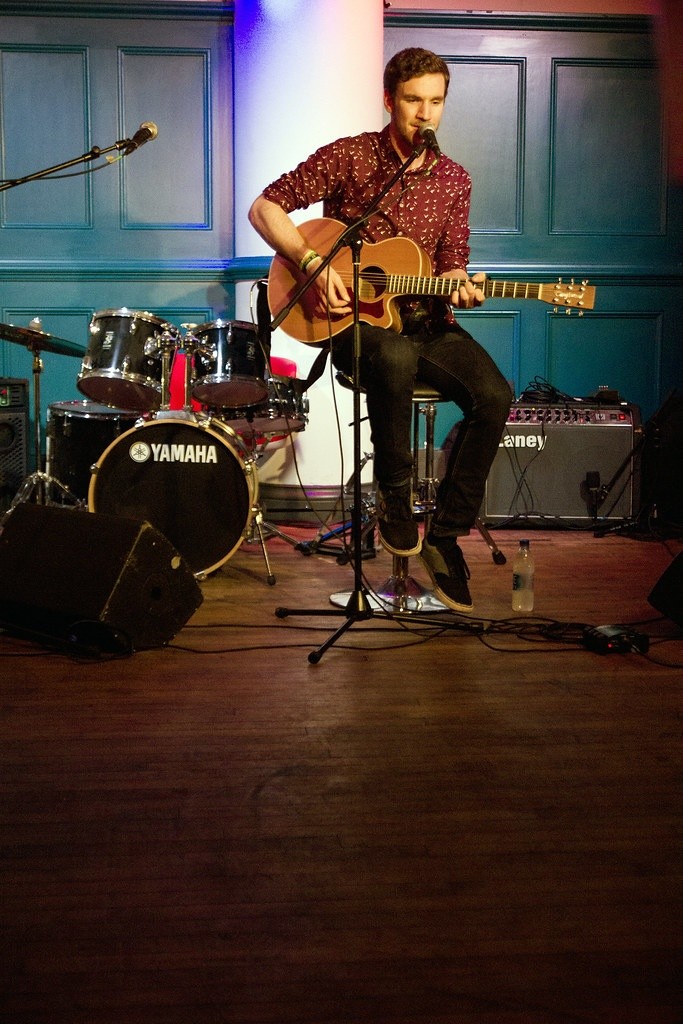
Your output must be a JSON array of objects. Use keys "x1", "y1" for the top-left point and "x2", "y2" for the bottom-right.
[{"x1": 1, "y1": 323, "x2": 89, "y2": 358}]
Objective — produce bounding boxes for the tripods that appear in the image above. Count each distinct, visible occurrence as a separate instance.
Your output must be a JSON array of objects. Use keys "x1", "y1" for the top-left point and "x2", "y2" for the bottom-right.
[
  {"x1": 269, "y1": 145, "x2": 483, "y2": 665},
  {"x1": 0, "y1": 339, "x2": 85, "y2": 535}
]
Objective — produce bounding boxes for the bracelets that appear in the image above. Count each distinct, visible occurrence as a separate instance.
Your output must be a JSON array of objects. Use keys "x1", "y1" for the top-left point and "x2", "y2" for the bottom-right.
[{"x1": 299, "y1": 249, "x2": 321, "y2": 275}]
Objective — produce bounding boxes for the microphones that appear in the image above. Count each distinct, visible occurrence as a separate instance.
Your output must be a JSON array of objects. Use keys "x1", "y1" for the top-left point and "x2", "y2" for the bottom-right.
[
  {"x1": 585, "y1": 470, "x2": 600, "y2": 522},
  {"x1": 123, "y1": 122, "x2": 157, "y2": 155},
  {"x1": 419, "y1": 122, "x2": 442, "y2": 158}
]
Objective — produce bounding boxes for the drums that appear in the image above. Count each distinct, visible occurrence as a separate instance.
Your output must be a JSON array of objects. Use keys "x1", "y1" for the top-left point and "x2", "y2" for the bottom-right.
[
  {"x1": 86, "y1": 410, "x2": 261, "y2": 580},
  {"x1": 74, "y1": 307, "x2": 181, "y2": 413},
  {"x1": 186, "y1": 318, "x2": 270, "y2": 411},
  {"x1": 44, "y1": 400, "x2": 143, "y2": 511},
  {"x1": 212, "y1": 375, "x2": 308, "y2": 436}
]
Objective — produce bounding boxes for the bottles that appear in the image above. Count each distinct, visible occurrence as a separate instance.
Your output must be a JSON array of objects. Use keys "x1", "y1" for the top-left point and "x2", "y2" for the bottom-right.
[{"x1": 512, "y1": 538, "x2": 534, "y2": 611}]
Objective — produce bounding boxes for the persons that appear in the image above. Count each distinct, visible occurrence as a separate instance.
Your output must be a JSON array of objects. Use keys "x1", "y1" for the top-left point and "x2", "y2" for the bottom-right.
[{"x1": 248, "y1": 48, "x2": 512, "y2": 615}]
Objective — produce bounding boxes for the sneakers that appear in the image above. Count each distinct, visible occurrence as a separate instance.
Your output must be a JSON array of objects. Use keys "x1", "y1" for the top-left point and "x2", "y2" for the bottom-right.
[
  {"x1": 420, "y1": 533, "x2": 474, "y2": 614},
  {"x1": 374, "y1": 480, "x2": 422, "y2": 557}
]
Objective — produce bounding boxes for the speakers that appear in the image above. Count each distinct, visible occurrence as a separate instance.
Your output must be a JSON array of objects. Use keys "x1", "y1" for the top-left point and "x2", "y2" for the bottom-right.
[
  {"x1": 647, "y1": 550, "x2": 683, "y2": 627},
  {"x1": 0, "y1": 501, "x2": 205, "y2": 656},
  {"x1": 478, "y1": 402, "x2": 644, "y2": 529}
]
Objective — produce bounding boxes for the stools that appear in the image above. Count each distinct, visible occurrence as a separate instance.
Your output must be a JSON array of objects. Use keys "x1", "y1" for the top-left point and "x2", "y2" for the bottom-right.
[{"x1": 336, "y1": 372, "x2": 459, "y2": 623}]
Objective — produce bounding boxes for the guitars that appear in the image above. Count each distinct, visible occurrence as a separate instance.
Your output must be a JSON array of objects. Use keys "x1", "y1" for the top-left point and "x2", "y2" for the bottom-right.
[{"x1": 265, "y1": 217, "x2": 596, "y2": 351}]
